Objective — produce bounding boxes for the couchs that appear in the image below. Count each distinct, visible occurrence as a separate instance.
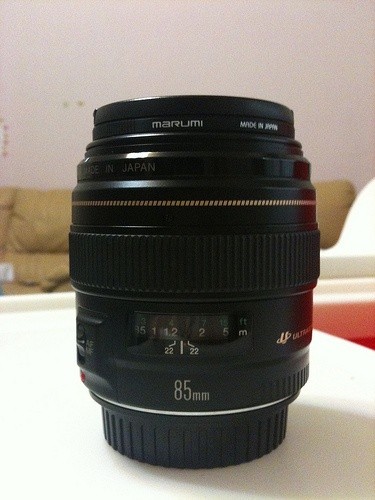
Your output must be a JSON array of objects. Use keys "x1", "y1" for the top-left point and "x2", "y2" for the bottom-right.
[{"x1": 0, "y1": 180, "x2": 356, "y2": 294}]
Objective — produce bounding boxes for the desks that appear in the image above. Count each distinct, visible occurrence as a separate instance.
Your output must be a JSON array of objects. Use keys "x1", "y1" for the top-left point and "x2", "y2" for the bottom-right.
[{"x1": 0, "y1": 291, "x2": 375, "y2": 500}]
